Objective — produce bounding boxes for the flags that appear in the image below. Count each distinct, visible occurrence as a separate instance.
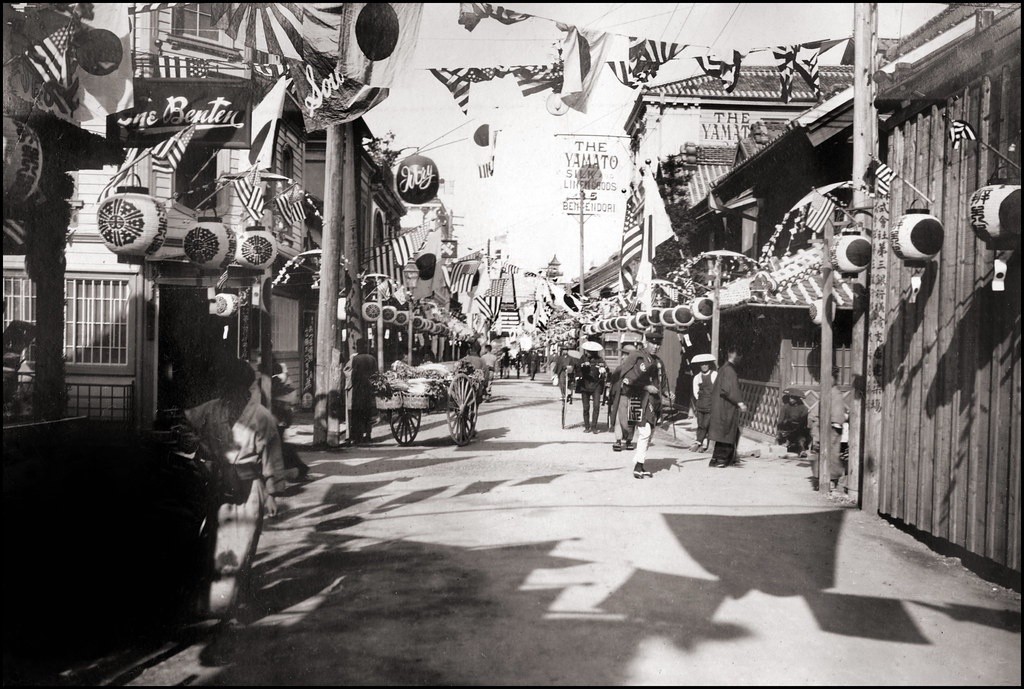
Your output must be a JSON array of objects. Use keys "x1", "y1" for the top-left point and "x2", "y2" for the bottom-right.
[
  {"x1": 529, "y1": 204, "x2": 677, "y2": 330},
  {"x1": 378, "y1": 229, "x2": 519, "y2": 327},
  {"x1": 23, "y1": 3, "x2": 977, "y2": 236}
]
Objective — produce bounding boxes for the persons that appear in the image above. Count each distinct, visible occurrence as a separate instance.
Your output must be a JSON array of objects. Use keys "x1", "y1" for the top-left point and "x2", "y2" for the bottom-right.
[
  {"x1": 830, "y1": 368, "x2": 845, "y2": 489},
  {"x1": 775, "y1": 388, "x2": 809, "y2": 457},
  {"x1": 154, "y1": 357, "x2": 286, "y2": 663},
  {"x1": 482, "y1": 340, "x2": 746, "y2": 476},
  {"x1": 340, "y1": 338, "x2": 453, "y2": 445}
]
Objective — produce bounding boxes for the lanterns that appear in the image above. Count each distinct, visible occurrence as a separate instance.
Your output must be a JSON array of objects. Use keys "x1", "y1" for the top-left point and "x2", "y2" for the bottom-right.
[
  {"x1": 236, "y1": 227, "x2": 277, "y2": 274},
  {"x1": 183, "y1": 216, "x2": 237, "y2": 274},
  {"x1": 362, "y1": 301, "x2": 470, "y2": 343},
  {"x1": 96, "y1": 186, "x2": 168, "y2": 263},
  {"x1": 968, "y1": 178, "x2": 1021, "y2": 250},
  {"x1": 835, "y1": 230, "x2": 871, "y2": 279},
  {"x1": 548, "y1": 295, "x2": 714, "y2": 346},
  {"x1": 892, "y1": 208, "x2": 944, "y2": 268}
]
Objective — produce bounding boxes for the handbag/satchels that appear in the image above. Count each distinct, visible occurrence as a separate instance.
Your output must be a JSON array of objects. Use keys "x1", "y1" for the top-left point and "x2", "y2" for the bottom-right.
[{"x1": 551, "y1": 374, "x2": 559, "y2": 386}]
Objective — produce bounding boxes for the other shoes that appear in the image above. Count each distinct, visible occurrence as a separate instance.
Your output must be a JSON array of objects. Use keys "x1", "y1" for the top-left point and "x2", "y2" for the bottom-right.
[
  {"x1": 699, "y1": 447, "x2": 704, "y2": 453},
  {"x1": 613, "y1": 441, "x2": 621, "y2": 451},
  {"x1": 710, "y1": 459, "x2": 726, "y2": 467},
  {"x1": 295, "y1": 465, "x2": 310, "y2": 483},
  {"x1": 690, "y1": 441, "x2": 700, "y2": 451},
  {"x1": 627, "y1": 441, "x2": 635, "y2": 449},
  {"x1": 634, "y1": 470, "x2": 653, "y2": 478}
]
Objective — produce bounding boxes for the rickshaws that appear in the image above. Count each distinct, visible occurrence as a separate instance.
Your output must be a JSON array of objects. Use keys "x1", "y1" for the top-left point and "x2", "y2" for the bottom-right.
[
  {"x1": 374, "y1": 341, "x2": 490, "y2": 447},
  {"x1": 560, "y1": 365, "x2": 614, "y2": 430},
  {"x1": 498, "y1": 346, "x2": 521, "y2": 379}
]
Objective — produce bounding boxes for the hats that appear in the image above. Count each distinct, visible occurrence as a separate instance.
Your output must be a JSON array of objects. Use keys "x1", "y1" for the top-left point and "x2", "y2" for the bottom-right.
[
  {"x1": 617, "y1": 344, "x2": 637, "y2": 355},
  {"x1": 582, "y1": 341, "x2": 603, "y2": 352},
  {"x1": 691, "y1": 354, "x2": 716, "y2": 363},
  {"x1": 645, "y1": 333, "x2": 663, "y2": 343},
  {"x1": 784, "y1": 388, "x2": 806, "y2": 400}
]
